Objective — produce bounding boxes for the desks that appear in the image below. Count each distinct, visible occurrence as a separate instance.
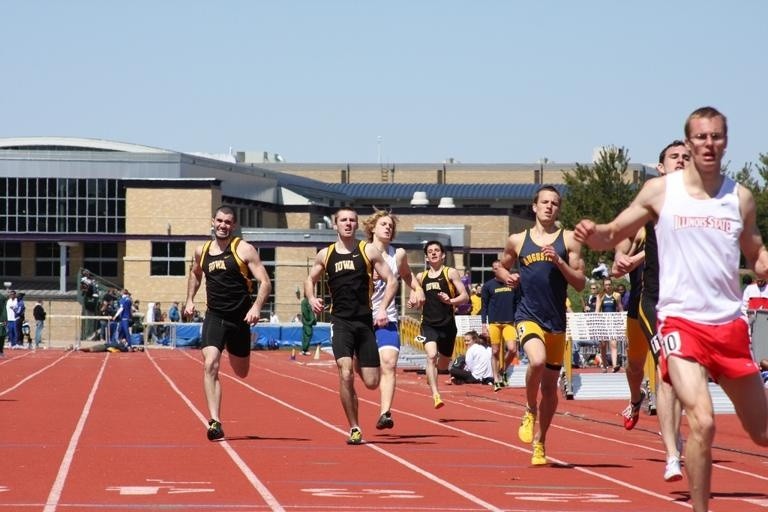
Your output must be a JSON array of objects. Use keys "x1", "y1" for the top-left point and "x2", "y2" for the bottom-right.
[{"x1": 129, "y1": 322, "x2": 332, "y2": 347}]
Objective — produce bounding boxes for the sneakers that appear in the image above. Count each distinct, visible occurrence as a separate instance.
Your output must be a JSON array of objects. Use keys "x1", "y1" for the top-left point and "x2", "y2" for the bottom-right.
[
  {"x1": 207, "y1": 419, "x2": 223, "y2": 440},
  {"x1": 434, "y1": 394, "x2": 443, "y2": 408},
  {"x1": 622, "y1": 388, "x2": 646, "y2": 430},
  {"x1": 376, "y1": 411, "x2": 393, "y2": 429},
  {"x1": 531, "y1": 442, "x2": 547, "y2": 465},
  {"x1": 664, "y1": 454, "x2": 682, "y2": 481},
  {"x1": 347, "y1": 428, "x2": 361, "y2": 444},
  {"x1": 518, "y1": 412, "x2": 535, "y2": 443}
]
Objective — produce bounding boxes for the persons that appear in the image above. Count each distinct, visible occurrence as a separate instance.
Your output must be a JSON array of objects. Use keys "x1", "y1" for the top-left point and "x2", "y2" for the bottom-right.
[
  {"x1": 493, "y1": 183, "x2": 586, "y2": 467},
  {"x1": 0, "y1": 290, "x2": 47, "y2": 354},
  {"x1": 294, "y1": 313, "x2": 301, "y2": 323},
  {"x1": 270, "y1": 311, "x2": 279, "y2": 323},
  {"x1": 448, "y1": 258, "x2": 518, "y2": 393},
  {"x1": 303, "y1": 205, "x2": 399, "y2": 446},
  {"x1": 361, "y1": 208, "x2": 427, "y2": 430},
  {"x1": 184, "y1": 205, "x2": 273, "y2": 441},
  {"x1": 572, "y1": 108, "x2": 768, "y2": 511},
  {"x1": 300, "y1": 296, "x2": 316, "y2": 357},
  {"x1": 80, "y1": 268, "x2": 204, "y2": 352},
  {"x1": 406, "y1": 240, "x2": 470, "y2": 408}
]
